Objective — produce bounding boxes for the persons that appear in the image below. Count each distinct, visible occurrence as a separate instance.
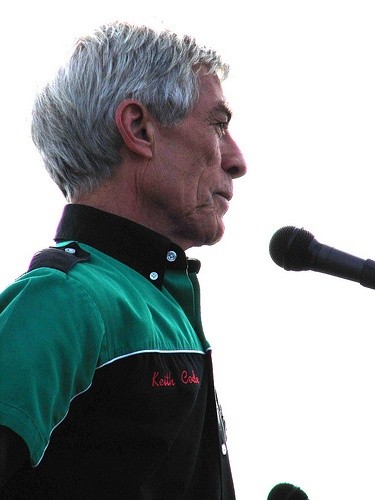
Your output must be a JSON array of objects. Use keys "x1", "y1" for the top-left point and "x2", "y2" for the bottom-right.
[{"x1": 0, "y1": 20, "x2": 247, "y2": 500}]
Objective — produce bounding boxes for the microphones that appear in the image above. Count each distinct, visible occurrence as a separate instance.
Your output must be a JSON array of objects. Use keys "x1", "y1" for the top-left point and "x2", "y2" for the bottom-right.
[
  {"x1": 266, "y1": 483, "x2": 309, "y2": 500},
  {"x1": 269, "y1": 226, "x2": 375, "y2": 290}
]
